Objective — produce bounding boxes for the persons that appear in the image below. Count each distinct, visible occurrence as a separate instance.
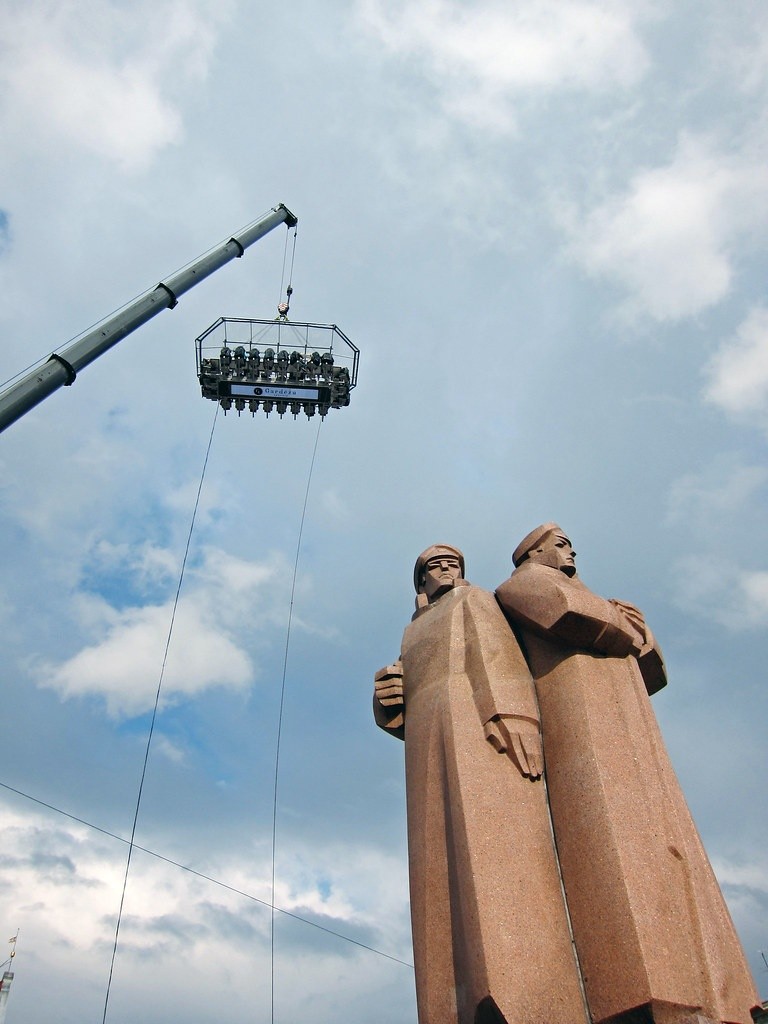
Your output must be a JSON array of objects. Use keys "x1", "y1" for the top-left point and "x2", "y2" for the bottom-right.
[
  {"x1": 369, "y1": 539, "x2": 589, "y2": 1024},
  {"x1": 493, "y1": 519, "x2": 767, "y2": 1024}
]
